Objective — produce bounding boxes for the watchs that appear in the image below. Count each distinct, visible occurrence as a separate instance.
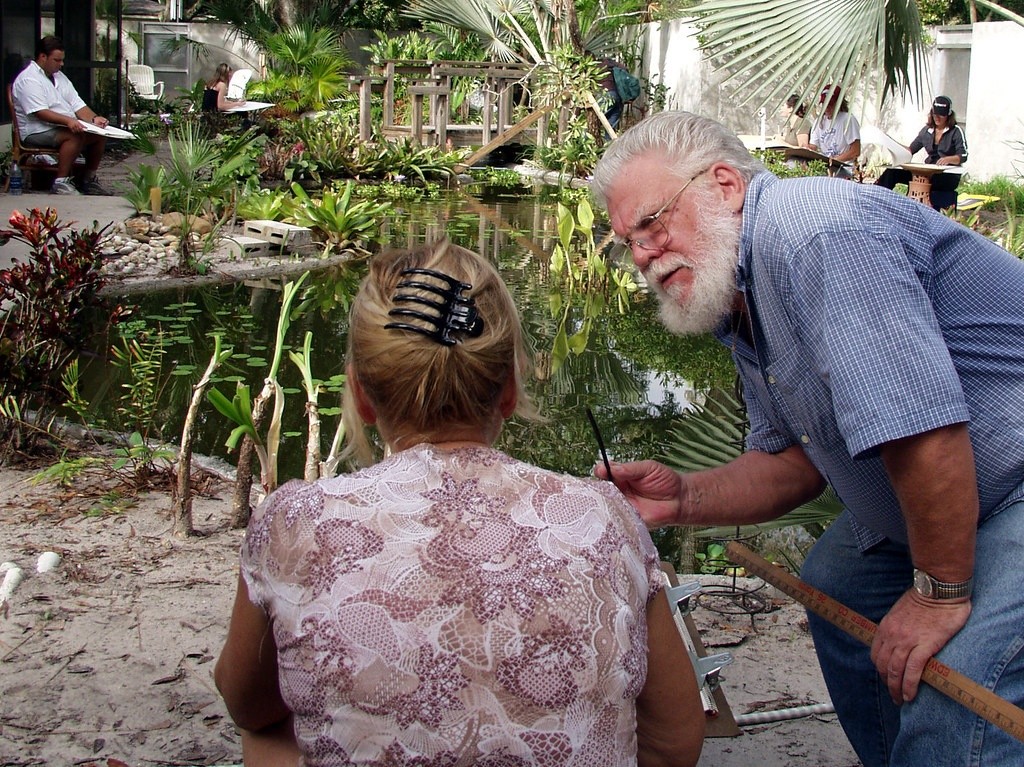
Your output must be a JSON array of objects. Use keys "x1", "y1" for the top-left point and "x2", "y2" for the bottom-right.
[{"x1": 912, "y1": 568, "x2": 974, "y2": 598}]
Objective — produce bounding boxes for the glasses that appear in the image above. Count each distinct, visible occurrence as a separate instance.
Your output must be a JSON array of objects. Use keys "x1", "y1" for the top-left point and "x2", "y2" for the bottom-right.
[
  {"x1": 609, "y1": 167, "x2": 711, "y2": 274},
  {"x1": 932, "y1": 114, "x2": 947, "y2": 118}
]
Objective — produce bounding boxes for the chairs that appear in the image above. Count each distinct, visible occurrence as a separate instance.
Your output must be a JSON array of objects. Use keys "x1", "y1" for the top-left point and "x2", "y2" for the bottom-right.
[
  {"x1": 1, "y1": 83, "x2": 60, "y2": 192},
  {"x1": 224, "y1": 69, "x2": 253, "y2": 102},
  {"x1": 127, "y1": 64, "x2": 165, "y2": 101},
  {"x1": 900, "y1": 162, "x2": 960, "y2": 209}
]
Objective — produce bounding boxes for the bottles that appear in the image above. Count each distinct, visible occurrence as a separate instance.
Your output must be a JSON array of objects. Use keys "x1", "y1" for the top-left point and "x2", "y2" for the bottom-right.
[{"x1": 9, "y1": 160, "x2": 23, "y2": 195}]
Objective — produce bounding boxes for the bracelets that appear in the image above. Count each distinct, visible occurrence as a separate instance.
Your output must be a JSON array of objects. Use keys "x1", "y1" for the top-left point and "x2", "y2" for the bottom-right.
[{"x1": 92, "y1": 115, "x2": 97, "y2": 123}]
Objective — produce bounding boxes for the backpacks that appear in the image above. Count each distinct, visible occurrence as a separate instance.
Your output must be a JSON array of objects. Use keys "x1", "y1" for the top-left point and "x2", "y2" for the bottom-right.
[{"x1": 612, "y1": 66, "x2": 640, "y2": 104}]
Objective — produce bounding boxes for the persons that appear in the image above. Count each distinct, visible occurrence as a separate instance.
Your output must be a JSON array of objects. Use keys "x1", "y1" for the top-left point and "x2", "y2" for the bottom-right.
[
  {"x1": 809, "y1": 83, "x2": 862, "y2": 180},
  {"x1": 875, "y1": 95, "x2": 968, "y2": 211},
  {"x1": 584, "y1": 50, "x2": 627, "y2": 139},
  {"x1": 589, "y1": 108, "x2": 1024, "y2": 767},
  {"x1": 12, "y1": 35, "x2": 113, "y2": 197},
  {"x1": 214, "y1": 229, "x2": 706, "y2": 767},
  {"x1": 775, "y1": 92, "x2": 812, "y2": 169},
  {"x1": 201, "y1": 64, "x2": 252, "y2": 130}
]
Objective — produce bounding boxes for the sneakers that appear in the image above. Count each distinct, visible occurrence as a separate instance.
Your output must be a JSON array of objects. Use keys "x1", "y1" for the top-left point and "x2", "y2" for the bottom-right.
[
  {"x1": 51, "y1": 176, "x2": 84, "y2": 195},
  {"x1": 82, "y1": 175, "x2": 116, "y2": 196}
]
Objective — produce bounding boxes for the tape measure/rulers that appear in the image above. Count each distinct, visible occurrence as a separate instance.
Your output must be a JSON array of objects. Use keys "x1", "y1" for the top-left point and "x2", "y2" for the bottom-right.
[{"x1": 723, "y1": 540, "x2": 1024, "y2": 741}]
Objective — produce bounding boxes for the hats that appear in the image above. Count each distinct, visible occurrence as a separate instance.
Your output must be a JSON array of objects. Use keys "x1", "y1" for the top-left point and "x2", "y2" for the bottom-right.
[
  {"x1": 932, "y1": 95, "x2": 953, "y2": 116},
  {"x1": 819, "y1": 84, "x2": 840, "y2": 104}
]
